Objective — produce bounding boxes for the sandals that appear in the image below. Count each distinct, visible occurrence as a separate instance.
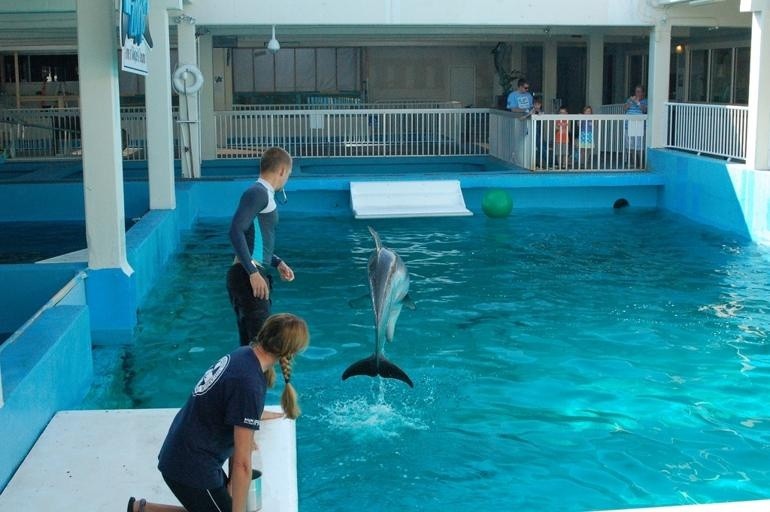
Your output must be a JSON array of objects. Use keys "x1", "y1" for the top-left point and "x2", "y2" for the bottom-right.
[{"x1": 127, "y1": 495, "x2": 146, "y2": 512}]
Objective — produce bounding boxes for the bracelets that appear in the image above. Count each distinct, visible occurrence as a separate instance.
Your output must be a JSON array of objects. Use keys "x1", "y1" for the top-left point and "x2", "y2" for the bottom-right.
[
  {"x1": 637, "y1": 102, "x2": 641, "y2": 105},
  {"x1": 629, "y1": 101, "x2": 632, "y2": 104}
]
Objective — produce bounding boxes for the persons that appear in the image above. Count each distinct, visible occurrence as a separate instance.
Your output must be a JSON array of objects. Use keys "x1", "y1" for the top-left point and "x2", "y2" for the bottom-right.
[
  {"x1": 622, "y1": 84, "x2": 647, "y2": 168},
  {"x1": 521, "y1": 99, "x2": 550, "y2": 167},
  {"x1": 123, "y1": 309, "x2": 312, "y2": 511},
  {"x1": 575, "y1": 107, "x2": 595, "y2": 170},
  {"x1": 227, "y1": 145, "x2": 297, "y2": 348},
  {"x1": 506, "y1": 78, "x2": 536, "y2": 114},
  {"x1": 553, "y1": 105, "x2": 570, "y2": 170}
]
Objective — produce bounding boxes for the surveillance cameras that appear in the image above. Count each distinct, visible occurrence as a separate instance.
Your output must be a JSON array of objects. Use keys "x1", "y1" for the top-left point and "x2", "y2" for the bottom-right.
[{"x1": 267, "y1": 37, "x2": 281, "y2": 55}]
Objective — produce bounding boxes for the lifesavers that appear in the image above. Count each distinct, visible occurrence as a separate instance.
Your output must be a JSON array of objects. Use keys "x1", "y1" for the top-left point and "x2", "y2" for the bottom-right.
[{"x1": 171, "y1": 63, "x2": 204, "y2": 96}]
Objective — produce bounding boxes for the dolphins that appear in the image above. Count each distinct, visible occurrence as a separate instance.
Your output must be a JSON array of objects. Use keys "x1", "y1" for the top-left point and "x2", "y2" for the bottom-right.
[{"x1": 338, "y1": 223, "x2": 417, "y2": 390}]
